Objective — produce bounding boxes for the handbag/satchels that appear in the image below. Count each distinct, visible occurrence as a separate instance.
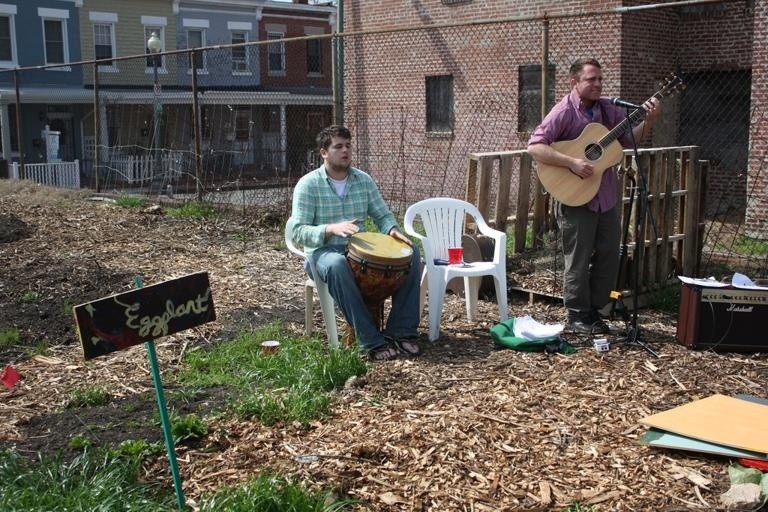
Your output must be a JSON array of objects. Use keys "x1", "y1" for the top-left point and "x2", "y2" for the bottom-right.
[{"x1": 490, "y1": 318, "x2": 576, "y2": 353}]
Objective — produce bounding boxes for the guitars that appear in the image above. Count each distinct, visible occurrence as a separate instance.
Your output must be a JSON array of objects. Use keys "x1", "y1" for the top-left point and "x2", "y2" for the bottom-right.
[{"x1": 535, "y1": 68, "x2": 689, "y2": 209}]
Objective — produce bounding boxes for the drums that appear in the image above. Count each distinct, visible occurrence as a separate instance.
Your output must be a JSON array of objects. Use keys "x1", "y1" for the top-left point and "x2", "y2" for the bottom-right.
[{"x1": 341, "y1": 231, "x2": 415, "y2": 347}]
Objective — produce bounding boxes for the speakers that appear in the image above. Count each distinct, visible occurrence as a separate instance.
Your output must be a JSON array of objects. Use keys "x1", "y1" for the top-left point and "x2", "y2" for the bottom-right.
[{"x1": 678, "y1": 282, "x2": 768, "y2": 354}]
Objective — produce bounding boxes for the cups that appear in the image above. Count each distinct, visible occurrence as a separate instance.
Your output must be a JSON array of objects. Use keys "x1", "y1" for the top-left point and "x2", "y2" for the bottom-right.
[
  {"x1": 262, "y1": 340, "x2": 280, "y2": 354},
  {"x1": 447, "y1": 247, "x2": 464, "y2": 264}
]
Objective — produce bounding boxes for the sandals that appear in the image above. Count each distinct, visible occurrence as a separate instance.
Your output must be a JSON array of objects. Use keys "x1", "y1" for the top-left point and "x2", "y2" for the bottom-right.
[
  {"x1": 369, "y1": 344, "x2": 398, "y2": 362},
  {"x1": 386, "y1": 336, "x2": 421, "y2": 356}
]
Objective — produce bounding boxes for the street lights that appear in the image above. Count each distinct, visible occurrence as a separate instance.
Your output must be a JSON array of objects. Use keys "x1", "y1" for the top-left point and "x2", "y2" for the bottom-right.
[{"x1": 148, "y1": 31, "x2": 163, "y2": 175}]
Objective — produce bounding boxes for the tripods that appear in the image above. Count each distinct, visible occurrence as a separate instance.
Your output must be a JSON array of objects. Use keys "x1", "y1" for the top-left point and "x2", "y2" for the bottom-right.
[{"x1": 587, "y1": 109, "x2": 662, "y2": 360}]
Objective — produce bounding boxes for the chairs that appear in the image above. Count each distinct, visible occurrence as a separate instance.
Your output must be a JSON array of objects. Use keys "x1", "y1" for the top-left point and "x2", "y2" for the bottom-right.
[
  {"x1": 403, "y1": 198, "x2": 508, "y2": 343},
  {"x1": 284, "y1": 218, "x2": 339, "y2": 347}
]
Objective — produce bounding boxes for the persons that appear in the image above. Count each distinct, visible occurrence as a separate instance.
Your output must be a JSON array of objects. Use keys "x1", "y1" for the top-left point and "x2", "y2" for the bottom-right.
[
  {"x1": 527, "y1": 60, "x2": 662, "y2": 335},
  {"x1": 292, "y1": 124, "x2": 420, "y2": 361}
]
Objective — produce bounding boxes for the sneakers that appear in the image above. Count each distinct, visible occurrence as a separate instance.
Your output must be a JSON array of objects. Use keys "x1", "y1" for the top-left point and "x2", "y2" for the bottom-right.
[
  {"x1": 566, "y1": 317, "x2": 607, "y2": 335},
  {"x1": 610, "y1": 311, "x2": 629, "y2": 320}
]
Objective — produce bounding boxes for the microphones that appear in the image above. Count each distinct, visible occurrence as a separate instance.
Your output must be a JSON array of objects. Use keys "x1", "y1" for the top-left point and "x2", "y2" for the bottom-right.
[{"x1": 610, "y1": 98, "x2": 639, "y2": 109}]
